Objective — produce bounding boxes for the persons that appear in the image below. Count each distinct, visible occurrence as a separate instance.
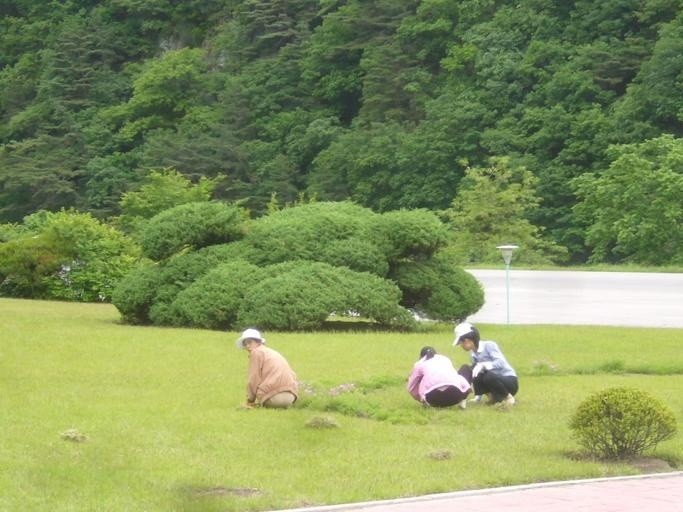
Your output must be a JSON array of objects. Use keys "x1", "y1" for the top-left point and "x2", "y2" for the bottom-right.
[
  {"x1": 406, "y1": 345, "x2": 473, "y2": 410},
  {"x1": 236, "y1": 328, "x2": 298, "y2": 409},
  {"x1": 450, "y1": 322, "x2": 519, "y2": 410}
]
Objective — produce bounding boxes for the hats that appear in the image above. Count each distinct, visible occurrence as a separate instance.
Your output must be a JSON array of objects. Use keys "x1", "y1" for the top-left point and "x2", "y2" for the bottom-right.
[
  {"x1": 237, "y1": 328, "x2": 266, "y2": 348},
  {"x1": 452, "y1": 322, "x2": 473, "y2": 347}
]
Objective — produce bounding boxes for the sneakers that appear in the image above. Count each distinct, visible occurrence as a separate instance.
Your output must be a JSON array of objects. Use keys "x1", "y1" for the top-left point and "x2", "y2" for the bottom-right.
[{"x1": 503, "y1": 395, "x2": 515, "y2": 405}]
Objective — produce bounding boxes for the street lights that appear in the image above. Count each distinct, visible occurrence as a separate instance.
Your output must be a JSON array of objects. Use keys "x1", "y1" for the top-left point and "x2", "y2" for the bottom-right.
[{"x1": 496, "y1": 245, "x2": 519, "y2": 324}]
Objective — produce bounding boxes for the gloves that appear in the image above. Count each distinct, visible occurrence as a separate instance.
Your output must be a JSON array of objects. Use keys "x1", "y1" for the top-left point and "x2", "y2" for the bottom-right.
[{"x1": 472, "y1": 363, "x2": 483, "y2": 379}]
